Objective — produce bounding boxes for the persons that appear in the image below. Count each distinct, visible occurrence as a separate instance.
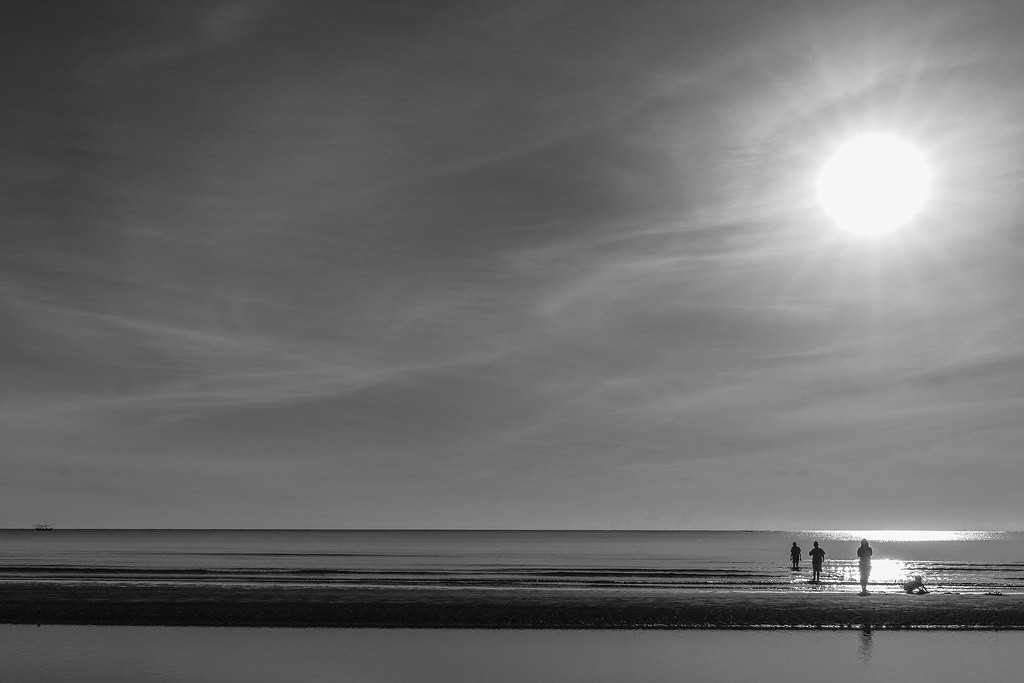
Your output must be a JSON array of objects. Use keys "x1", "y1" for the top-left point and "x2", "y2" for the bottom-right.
[
  {"x1": 857, "y1": 538, "x2": 872, "y2": 595},
  {"x1": 809, "y1": 541, "x2": 826, "y2": 583},
  {"x1": 790, "y1": 541, "x2": 801, "y2": 569},
  {"x1": 904, "y1": 575, "x2": 928, "y2": 594}
]
